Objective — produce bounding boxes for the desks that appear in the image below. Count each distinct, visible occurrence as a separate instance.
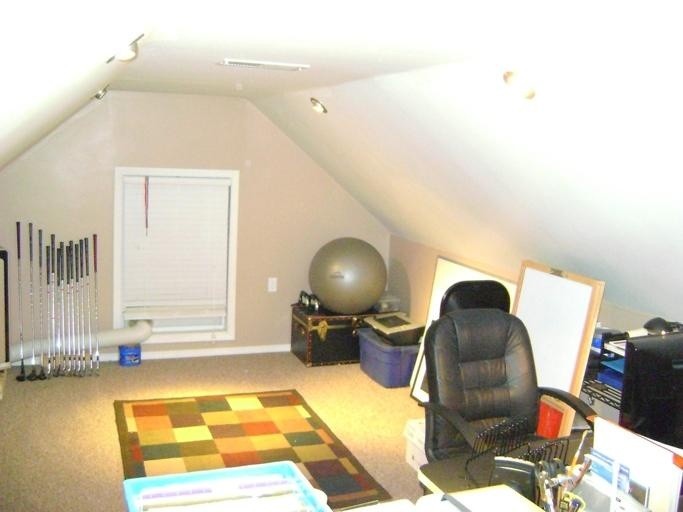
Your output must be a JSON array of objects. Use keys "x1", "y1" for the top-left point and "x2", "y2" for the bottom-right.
[{"x1": 417, "y1": 429, "x2": 594, "y2": 498}]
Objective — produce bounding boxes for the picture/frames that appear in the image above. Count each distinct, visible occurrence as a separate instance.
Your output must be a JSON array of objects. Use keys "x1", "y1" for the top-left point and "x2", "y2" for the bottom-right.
[{"x1": 410, "y1": 257, "x2": 607, "y2": 404}]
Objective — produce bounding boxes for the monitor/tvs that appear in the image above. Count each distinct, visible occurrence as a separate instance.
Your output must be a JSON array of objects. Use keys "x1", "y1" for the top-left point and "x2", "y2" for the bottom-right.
[{"x1": 619, "y1": 332, "x2": 683, "y2": 449}]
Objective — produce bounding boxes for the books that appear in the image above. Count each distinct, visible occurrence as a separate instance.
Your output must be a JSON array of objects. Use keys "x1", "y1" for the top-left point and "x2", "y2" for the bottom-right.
[{"x1": 563, "y1": 414, "x2": 683, "y2": 512}]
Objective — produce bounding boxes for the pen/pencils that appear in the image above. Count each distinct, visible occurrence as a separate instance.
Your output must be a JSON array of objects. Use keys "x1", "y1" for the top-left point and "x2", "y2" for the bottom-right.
[{"x1": 535, "y1": 458, "x2": 592, "y2": 512}]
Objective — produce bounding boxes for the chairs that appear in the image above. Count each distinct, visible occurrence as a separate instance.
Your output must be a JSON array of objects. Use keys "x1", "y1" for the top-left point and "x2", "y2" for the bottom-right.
[{"x1": 421, "y1": 308, "x2": 597, "y2": 461}]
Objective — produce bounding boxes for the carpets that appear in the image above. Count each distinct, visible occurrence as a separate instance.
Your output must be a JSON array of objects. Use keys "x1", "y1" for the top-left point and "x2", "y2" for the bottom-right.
[{"x1": 114, "y1": 389, "x2": 392, "y2": 510}]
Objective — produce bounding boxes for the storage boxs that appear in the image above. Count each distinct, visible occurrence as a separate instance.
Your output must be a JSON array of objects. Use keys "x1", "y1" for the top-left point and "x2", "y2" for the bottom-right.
[{"x1": 291, "y1": 304, "x2": 421, "y2": 388}]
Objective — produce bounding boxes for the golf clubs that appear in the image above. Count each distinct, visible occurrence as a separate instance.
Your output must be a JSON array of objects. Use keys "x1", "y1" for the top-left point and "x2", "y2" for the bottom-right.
[
  {"x1": 15, "y1": 221, "x2": 24, "y2": 381},
  {"x1": 26, "y1": 223, "x2": 99, "y2": 380}
]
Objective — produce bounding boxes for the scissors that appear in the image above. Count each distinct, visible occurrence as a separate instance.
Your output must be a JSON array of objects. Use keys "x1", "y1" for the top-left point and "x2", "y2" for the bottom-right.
[{"x1": 534, "y1": 457, "x2": 566, "y2": 479}]
